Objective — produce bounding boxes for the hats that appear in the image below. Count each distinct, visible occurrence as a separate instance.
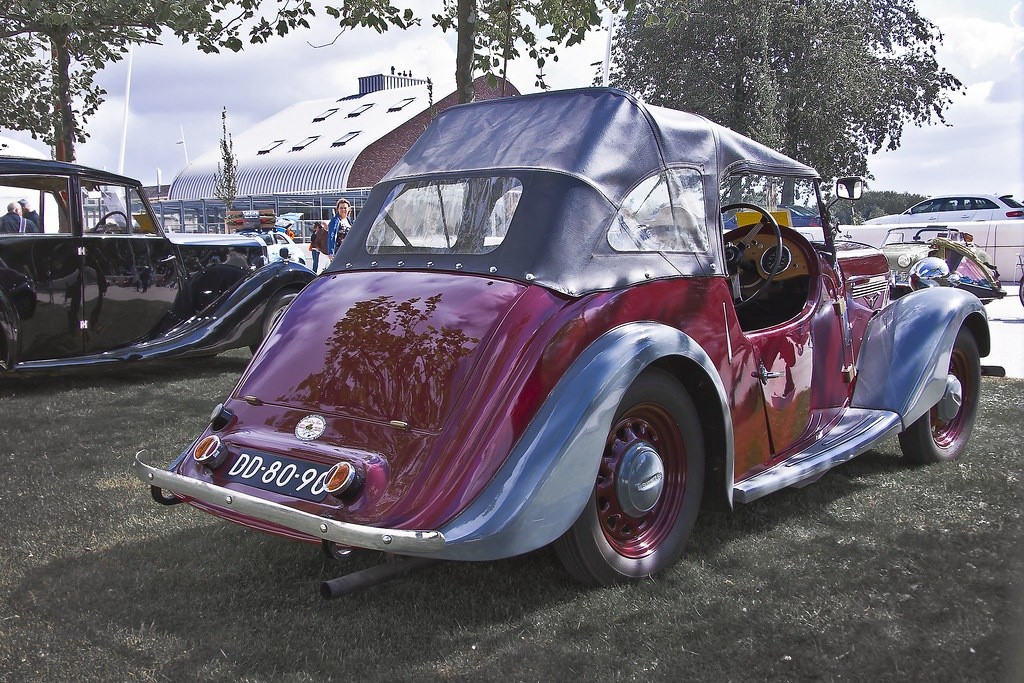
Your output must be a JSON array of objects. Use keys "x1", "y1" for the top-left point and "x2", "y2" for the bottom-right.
[{"x1": 17, "y1": 199, "x2": 28, "y2": 206}]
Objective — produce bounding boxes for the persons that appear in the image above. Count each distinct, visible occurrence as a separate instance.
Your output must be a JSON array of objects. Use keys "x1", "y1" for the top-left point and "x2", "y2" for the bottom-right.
[
  {"x1": 327, "y1": 199, "x2": 354, "y2": 262},
  {"x1": 0, "y1": 202, "x2": 38, "y2": 234},
  {"x1": 140, "y1": 265, "x2": 151, "y2": 293},
  {"x1": 309, "y1": 220, "x2": 330, "y2": 274},
  {"x1": 18, "y1": 199, "x2": 39, "y2": 226},
  {"x1": 211, "y1": 247, "x2": 249, "y2": 269}
]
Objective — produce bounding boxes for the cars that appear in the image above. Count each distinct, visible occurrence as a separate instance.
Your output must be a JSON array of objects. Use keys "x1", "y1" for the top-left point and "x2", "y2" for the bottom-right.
[
  {"x1": 724, "y1": 205, "x2": 822, "y2": 230},
  {"x1": 879, "y1": 225, "x2": 991, "y2": 301},
  {"x1": 859, "y1": 195, "x2": 1024, "y2": 225},
  {"x1": 0, "y1": 155, "x2": 319, "y2": 378},
  {"x1": 133, "y1": 87, "x2": 991, "y2": 589}
]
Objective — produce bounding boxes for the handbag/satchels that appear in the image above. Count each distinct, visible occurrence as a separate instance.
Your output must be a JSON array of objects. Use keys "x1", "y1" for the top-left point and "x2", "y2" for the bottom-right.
[
  {"x1": 313, "y1": 227, "x2": 329, "y2": 255},
  {"x1": 309, "y1": 243, "x2": 313, "y2": 251}
]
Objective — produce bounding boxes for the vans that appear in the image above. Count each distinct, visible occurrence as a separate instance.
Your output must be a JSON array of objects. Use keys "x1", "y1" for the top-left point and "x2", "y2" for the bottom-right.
[{"x1": 234, "y1": 232, "x2": 308, "y2": 268}]
